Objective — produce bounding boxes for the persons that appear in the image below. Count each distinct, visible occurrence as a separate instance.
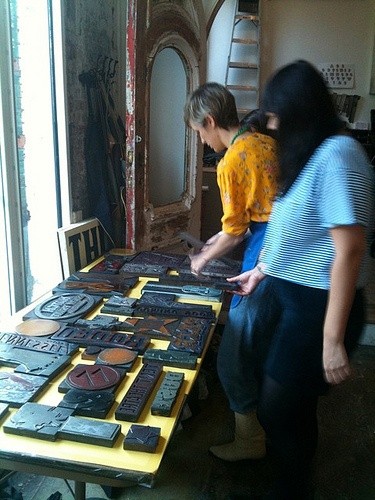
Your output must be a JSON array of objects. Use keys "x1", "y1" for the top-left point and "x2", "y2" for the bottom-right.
[
  {"x1": 240, "y1": 108, "x2": 272, "y2": 137},
  {"x1": 182, "y1": 81, "x2": 280, "y2": 460},
  {"x1": 225, "y1": 56, "x2": 374, "y2": 500}
]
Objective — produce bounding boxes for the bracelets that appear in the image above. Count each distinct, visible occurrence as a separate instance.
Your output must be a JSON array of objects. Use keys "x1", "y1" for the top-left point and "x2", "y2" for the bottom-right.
[{"x1": 256, "y1": 264, "x2": 268, "y2": 277}]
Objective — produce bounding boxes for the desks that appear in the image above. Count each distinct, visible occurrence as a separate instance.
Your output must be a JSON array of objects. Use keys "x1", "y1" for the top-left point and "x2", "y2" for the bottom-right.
[{"x1": 0, "y1": 248, "x2": 222, "y2": 500}]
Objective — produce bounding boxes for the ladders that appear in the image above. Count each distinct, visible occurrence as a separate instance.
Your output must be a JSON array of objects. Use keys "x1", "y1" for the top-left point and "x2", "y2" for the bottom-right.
[{"x1": 225, "y1": 0, "x2": 261, "y2": 116}]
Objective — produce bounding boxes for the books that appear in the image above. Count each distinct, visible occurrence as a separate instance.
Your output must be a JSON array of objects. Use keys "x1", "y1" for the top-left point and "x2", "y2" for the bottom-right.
[{"x1": 329, "y1": 93, "x2": 362, "y2": 123}]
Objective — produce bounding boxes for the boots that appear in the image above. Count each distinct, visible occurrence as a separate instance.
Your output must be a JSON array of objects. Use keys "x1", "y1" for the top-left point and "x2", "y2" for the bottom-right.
[{"x1": 208, "y1": 412, "x2": 266, "y2": 462}]
